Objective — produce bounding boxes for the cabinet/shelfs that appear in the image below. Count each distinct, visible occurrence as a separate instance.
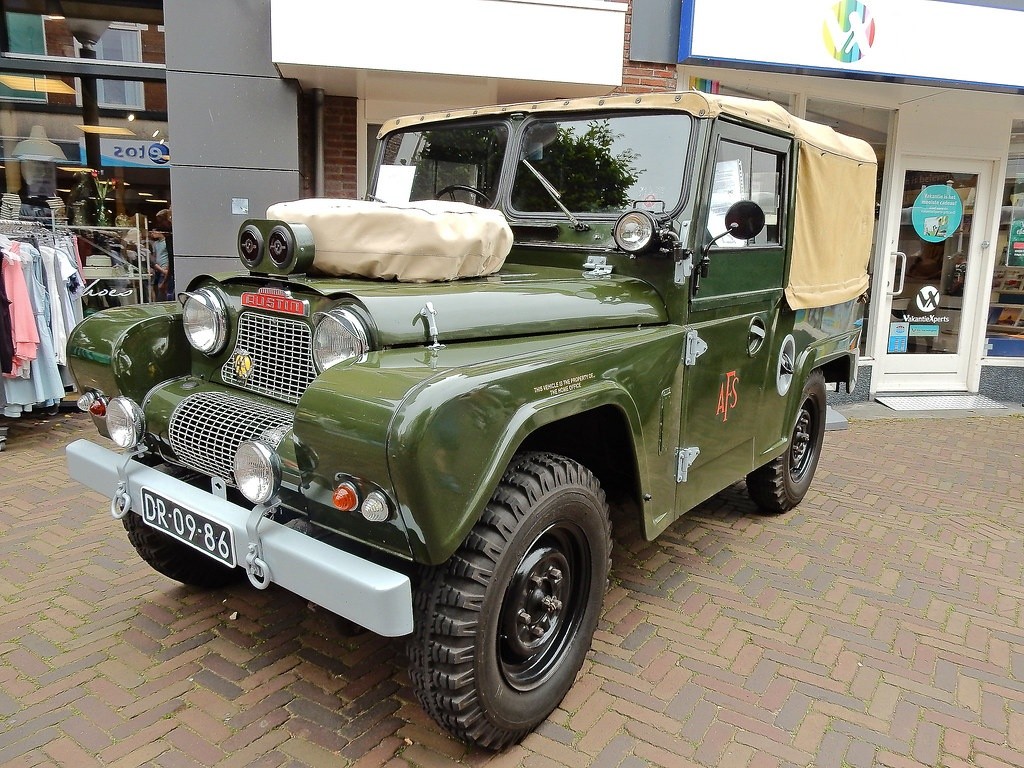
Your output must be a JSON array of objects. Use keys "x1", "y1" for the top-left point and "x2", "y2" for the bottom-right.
[
  {"x1": 51, "y1": 209, "x2": 153, "y2": 304},
  {"x1": 903, "y1": 188, "x2": 1024, "y2": 356}
]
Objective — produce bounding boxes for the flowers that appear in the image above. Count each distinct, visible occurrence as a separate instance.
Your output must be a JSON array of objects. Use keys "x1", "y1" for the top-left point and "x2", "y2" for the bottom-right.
[{"x1": 91, "y1": 169, "x2": 119, "y2": 219}]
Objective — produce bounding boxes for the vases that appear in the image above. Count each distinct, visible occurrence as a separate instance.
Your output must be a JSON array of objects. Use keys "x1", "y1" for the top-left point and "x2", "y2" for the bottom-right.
[{"x1": 96, "y1": 197, "x2": 112, "y2": 226}]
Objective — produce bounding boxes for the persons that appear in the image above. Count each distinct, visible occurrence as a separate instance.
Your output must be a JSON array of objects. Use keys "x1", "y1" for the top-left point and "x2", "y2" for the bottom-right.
[{"x1": 120, "y1": 225, "x2": 172, "y2": 305}]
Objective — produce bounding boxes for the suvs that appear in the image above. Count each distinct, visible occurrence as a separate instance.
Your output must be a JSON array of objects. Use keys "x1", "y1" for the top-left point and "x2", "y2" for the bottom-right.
[{"x1": 63, "y1": 89, "x2": 878, "y2": 755}]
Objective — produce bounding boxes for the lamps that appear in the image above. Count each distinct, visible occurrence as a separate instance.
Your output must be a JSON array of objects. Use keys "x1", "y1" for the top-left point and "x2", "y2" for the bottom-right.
[{"x1": 11, "y1": 71, "x2": 68, "y2": 160}]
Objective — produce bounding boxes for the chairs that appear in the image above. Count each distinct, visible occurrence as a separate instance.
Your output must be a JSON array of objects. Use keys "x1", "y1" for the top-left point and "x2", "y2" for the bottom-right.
[{"x1": 495, "y1": 160, "x2": 546, "y2": 212}]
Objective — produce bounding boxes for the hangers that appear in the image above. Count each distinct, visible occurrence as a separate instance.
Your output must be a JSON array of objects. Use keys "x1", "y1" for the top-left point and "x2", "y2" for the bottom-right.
[{"x1": 0, "y1": 218, "x2": 76, "y2": 246}]
